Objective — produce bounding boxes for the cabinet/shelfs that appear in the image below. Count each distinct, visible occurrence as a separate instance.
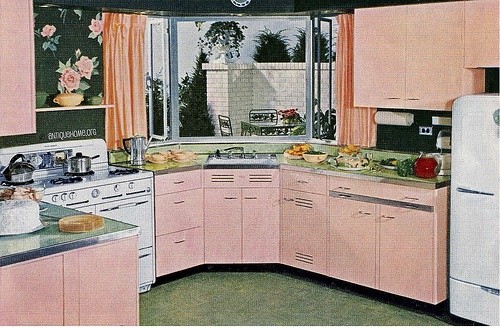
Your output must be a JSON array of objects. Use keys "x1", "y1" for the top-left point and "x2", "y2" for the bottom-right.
[
  {"x1": 280, "y1": 148, "x2": 450, "y2": 307},
  {"x1": 203, "y1": 152, "x2": 281, "y2": 267},
  {"x1": 153, "y1": 155, "x2": 204, "y2": 280}
]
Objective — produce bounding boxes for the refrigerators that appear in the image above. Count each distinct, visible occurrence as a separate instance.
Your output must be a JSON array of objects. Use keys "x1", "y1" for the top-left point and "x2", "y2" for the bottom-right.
[{"x1": 450, "y1": 93, "x2": 500, "y2": 325}]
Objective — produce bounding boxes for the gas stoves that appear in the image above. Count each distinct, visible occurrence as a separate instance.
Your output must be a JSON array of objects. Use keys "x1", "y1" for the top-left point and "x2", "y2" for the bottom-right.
[{"x1": 0, "y1": 139, "x2": 154, "y2": 210}]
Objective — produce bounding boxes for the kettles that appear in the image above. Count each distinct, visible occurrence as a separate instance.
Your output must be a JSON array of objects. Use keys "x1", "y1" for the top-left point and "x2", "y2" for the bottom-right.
[{"x1": 1, "y1": 153, "x2": 36, "y2": 185}]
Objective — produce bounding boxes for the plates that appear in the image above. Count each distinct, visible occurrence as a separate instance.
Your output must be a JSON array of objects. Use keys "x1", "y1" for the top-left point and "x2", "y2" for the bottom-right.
[
  {"x1": 337, "y1": 165, "x2": 370, "y2": 171},
  {"x1": 172, "y1": 158, "x2": 189, "y2": 163},
  {"x1": 165, "y1": 155, "x2": 174, "y2": 159},
  {"x1": 380, "y1": 164, "x2": 398, "y2": 170},
  {"x1": 149, "y1": 159, "x2": 167, "y2": 165}
]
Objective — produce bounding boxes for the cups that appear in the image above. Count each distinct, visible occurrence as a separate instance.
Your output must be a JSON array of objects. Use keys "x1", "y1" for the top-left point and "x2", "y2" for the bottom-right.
[
  {"x1": 183, "y1": 150, "x2": 197, "y2": 159},
  {"x1": 171, "y1": 150, "x2": 183, "y2": 157},
  {"x1": 175, "y1": 153, "x2": 187, "y2": 160},
  {"x1": 152, "y1": 153, "x2": 166, "y2": 161}
]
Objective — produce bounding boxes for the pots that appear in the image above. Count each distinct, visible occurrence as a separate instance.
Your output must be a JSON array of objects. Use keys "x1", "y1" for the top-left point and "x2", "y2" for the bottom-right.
[{"x1": 62, "y1": 152, "x2": 102, "y2": 174}]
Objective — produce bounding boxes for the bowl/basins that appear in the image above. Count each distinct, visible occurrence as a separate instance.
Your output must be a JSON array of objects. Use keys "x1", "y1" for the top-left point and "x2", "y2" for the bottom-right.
[
  {"x1": 0, "y1": 186, "x2": 45, "y2": 204},
  {"x1": 87, "y1": 95, "x2": 103, "y2": 105},
  {"x1": 53, "y1": 90, "x2": 84, "y2": 107},
  {"x1": 303, "y1": 152, "x2": 328, "y2": 163}
]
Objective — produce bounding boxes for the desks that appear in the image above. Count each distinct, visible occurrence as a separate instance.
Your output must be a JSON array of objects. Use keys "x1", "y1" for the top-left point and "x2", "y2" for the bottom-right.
[{"x1": 240, "y1": 120, "x2": 306, "y2": 135}]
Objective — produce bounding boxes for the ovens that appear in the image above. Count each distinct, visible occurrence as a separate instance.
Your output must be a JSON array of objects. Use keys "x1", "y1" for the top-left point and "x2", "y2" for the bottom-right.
[{"x1": 72, "y1": 193, "x2": 157, "y2": 295}]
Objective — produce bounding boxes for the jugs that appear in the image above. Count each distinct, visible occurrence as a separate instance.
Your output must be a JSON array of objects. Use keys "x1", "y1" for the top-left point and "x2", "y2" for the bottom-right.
[
  {"x1": 123, "y1": 135, "x2": 153, "y2": 166},
  {"x1": 413, "y1": 151, "x2": 443, "y2": 179}
]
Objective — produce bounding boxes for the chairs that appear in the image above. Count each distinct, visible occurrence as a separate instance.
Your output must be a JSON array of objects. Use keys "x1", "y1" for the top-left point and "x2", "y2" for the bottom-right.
[
  {"x1": 219, "y1": 115, "x2": 233, "y2": 136},
  {"x1": 248, "y1": 109, "x2": 279, "y2": 124}
]
